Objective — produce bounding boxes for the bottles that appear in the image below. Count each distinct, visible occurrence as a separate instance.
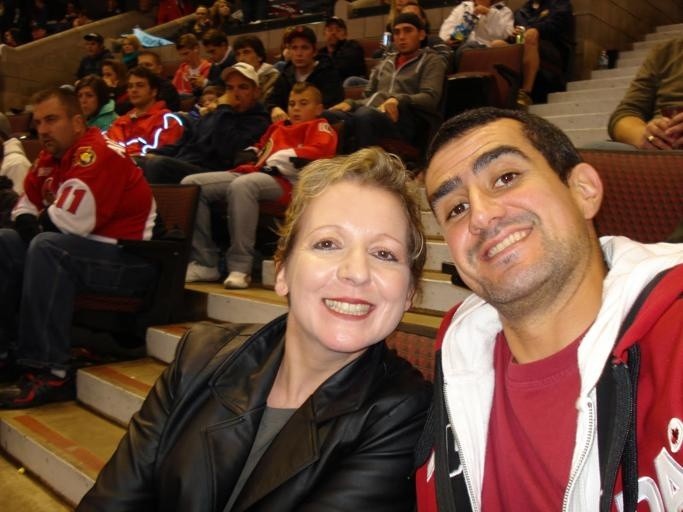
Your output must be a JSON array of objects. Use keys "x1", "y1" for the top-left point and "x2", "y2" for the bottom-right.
[
  {"x1": 515, "y1": 27, "x2": 524, "y2": 44},
  {"x1": 598, "y1": 49, "x2": 609, "y2": 70}
]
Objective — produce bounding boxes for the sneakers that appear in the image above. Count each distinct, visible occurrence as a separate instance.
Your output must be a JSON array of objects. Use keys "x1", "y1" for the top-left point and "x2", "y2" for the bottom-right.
[
  {"x1": 223, "y1": 268, "x2": 252, "y2": 290},
  {"x1": 182, "y1": 260, "x2": 220, "y2": 284},
  {"x1": 516, "y1": 88, "x2": 532, "y2": 107},
  {"x1": 1, "y1": 367, "x2": 78, "y2": 410}
]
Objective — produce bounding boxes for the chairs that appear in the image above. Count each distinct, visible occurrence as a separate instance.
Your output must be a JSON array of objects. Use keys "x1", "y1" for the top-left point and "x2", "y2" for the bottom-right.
[
  {"x1": 213, "y1": 196, "x2": 288, "y2": 218},
  {"x1": 329, "y1": 42, "x2": 525, "y2": 180},
  {"x1": 365, "y1": 321, "x2": 438, "y2": 383},
  {"x1": 543, "y1": 145, "x2": 680, "y2": 246},
  {"x1": 70, "y1": 183, "x2": 202, "y2": 313}
]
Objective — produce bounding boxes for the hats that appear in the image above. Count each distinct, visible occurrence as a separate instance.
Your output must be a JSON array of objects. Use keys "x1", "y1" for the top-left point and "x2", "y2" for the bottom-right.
[
  {"x1": 324, "y1": 15, "x2": 347, "y2": 29},
  {"x1": 287, "y1": 25, "x2": 317, "y2": 46},
  {"x1": 393, "y1": 12, "x2": 425, "y2": 33},
  {"x1": 83, "y1": 32, "x2": 103, "y2": 44},
  {"x1": 221, "y1": 61, "x2": 259, "y2": 88}
]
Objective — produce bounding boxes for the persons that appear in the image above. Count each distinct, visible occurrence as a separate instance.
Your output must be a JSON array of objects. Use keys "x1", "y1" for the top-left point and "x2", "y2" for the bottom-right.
[
  {"x1": 410, "y1": 108, "x2": 683, "y2": 512},
  {"x1": 70, "y1": 144, "x2": 433, "y2": 512},
  {"x1": 604, "y1": 37, "x2": 682, "y2": 150}
]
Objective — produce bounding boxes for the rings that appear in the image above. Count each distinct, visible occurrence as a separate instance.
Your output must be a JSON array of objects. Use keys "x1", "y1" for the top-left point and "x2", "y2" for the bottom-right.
[{"x1": 647, "y1": 134, "x2": 655, "y2": 141}]
